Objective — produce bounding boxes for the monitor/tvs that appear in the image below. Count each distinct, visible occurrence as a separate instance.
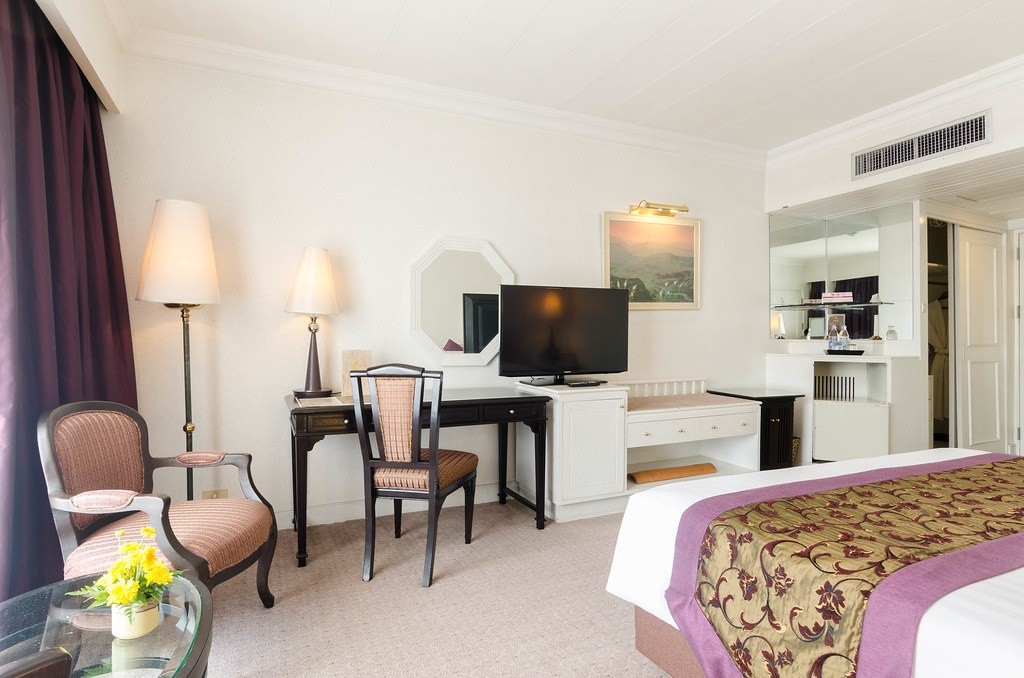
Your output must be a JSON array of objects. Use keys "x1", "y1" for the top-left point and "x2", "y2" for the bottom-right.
[
  {"x1": 463, "y1": 294, "x2": 499, "y2": 353},
  {"x1": 498, "y1": 284, "x2": 629, "y2": 387}
]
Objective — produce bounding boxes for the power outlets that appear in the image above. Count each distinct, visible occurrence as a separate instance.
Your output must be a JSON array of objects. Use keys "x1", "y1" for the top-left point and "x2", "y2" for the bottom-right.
[{"x1": 202, "y1": 488, "x2": 228, "y2": 500}]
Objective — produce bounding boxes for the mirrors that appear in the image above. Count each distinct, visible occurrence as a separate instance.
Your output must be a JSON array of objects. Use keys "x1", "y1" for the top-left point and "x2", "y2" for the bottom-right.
[
  {"x1": 768, "y1": 201, "x2": 913, "y2": 340},
  {"x1": 410, "y1": 236, "x2": 515, "y2": 367}
]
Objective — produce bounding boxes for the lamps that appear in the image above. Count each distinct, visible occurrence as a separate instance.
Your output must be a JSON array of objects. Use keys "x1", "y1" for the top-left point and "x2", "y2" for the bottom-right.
[
  {"x1": 630, "y1": 200, "x2": 689, "y2": 217},
  {"x1": 284, "y1": 247, "x2": 338, "y2": 398},
  {"x1": 135, "y1": 198, "x2": 223, "y2": 501}
]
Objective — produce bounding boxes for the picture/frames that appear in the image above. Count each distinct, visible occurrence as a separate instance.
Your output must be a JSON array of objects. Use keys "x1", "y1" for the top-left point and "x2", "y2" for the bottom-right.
[{"x1": 602, "y1": 211, "x2": 701, "y2": 310}]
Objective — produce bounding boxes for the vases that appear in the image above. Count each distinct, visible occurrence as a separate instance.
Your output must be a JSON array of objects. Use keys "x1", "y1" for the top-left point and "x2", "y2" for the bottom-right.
[{"x1": 112, "y1": 598, "x2": 160, "y2": 640}]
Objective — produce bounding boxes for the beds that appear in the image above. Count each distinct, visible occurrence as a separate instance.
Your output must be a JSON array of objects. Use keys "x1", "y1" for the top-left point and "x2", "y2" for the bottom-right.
[{"x1": 604, "y1": 447, "x2": 1024, "y2": 678}]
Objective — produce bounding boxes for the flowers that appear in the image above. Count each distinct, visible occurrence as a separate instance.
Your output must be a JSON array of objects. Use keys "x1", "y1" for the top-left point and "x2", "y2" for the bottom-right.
[{"x1": 65, "y1": 527, "x2": 191, "y2": 610}]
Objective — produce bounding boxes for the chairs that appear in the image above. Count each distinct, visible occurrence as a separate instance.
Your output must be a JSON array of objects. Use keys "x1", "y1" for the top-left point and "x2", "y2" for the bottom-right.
[
  {"x1": 350, "y1": 363, "x2": 479, "y2": 588},
  {"x1": 38, "y1": 400, "x2": 278, "y2": 608}
]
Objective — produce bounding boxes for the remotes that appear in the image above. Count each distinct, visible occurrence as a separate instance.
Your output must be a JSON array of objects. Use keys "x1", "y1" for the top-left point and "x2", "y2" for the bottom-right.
[{"x1": 567, "y1": 380, "x2": 600, "y2": 387}]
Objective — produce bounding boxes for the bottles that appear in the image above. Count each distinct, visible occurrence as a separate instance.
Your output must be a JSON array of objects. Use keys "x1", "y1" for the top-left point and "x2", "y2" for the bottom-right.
[
  {"x1": 829, "y1": 324, "x2": 838, "y2": 350},
  {"x1": 838, "y1": 325, "x2": 849, "y2": 350}
]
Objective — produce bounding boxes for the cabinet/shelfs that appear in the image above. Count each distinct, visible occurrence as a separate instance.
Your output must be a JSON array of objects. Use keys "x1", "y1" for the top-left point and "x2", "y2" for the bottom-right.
[
  {"x1": 627, "y1": 393, "x2": 763, "y2": 496},
  {"x1": 764, "y1": 353, "x2": 921, "y2": 465},
  {"x1": 515, "y1": 382, "x2": 630, "y2": 523},
  {"x1": 707, "y1": 388, "x2": 807, "y2": 471}
]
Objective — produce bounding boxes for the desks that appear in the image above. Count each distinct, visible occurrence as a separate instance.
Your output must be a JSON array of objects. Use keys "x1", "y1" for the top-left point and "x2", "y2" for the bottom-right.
[
  {"x1": 285, "y1": 386, "x2": 552, "y2": 568},
  {"x1": 0, "y1": 571, "x2": 213, "y2": 678}
]
